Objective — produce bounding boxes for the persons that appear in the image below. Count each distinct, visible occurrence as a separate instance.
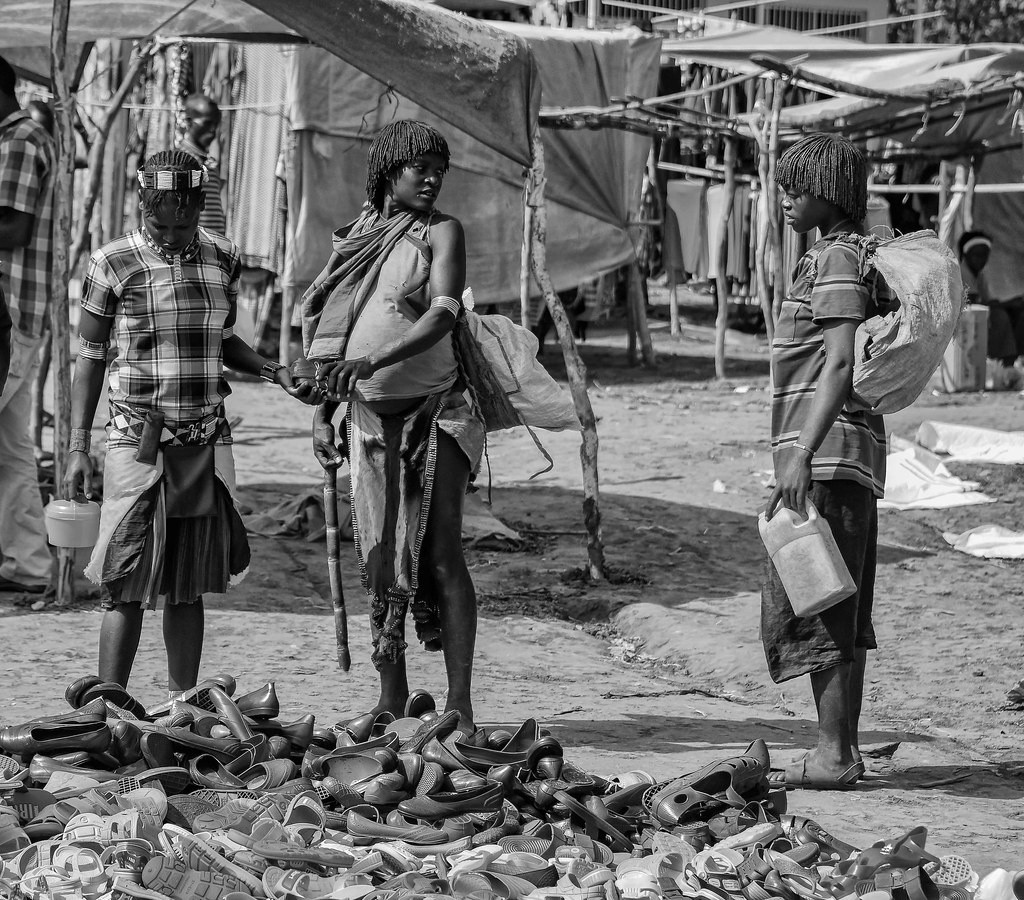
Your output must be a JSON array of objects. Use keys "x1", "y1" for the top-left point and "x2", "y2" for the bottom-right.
[
  {"x1": 304, "y1": 120, "x2": 486, "y2": 738},
  {"x1": 62, "y1": 151, "x2": 322, "y2": 703},
  {"x1": 957, "y1": 230, "x2": 1023, "y2": 388},
  {"x1": 0, "y1": 55, "x2": 72, "y2": 595},
  {"x1": 761, "y1": 133, "x2": 887, "y2": 788},
  {"x1": 173, "y1": 92, "x2": 225, "y2": 237}
]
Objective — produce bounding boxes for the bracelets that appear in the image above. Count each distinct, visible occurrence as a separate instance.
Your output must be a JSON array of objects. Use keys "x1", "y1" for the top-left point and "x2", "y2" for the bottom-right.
[
  {"x1": 260, "y1": 361, "x2": 286, "y2": 383},
  {"x1": 793, "y1": 442, "x2": 817, "y2": 456},
  {"x1": 70, "y1": 429, "x2": 91, "y2": 453},
  {"x1": 430, "y1": 294, "x2": 462, "y2": 321}
]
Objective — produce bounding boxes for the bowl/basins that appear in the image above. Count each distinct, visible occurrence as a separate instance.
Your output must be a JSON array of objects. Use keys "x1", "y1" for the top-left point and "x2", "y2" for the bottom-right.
[{"x1": 44, "y1": 499, "x2": 100, "y2": 548}]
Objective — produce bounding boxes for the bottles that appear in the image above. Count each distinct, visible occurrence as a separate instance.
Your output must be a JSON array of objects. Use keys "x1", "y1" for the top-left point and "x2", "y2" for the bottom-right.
[{"x1": 757, "y1": 494, "x2": 857, "y2": 618}]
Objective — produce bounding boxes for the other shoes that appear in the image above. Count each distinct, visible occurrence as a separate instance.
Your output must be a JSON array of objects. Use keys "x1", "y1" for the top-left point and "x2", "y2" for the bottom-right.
[
  {"x1": 0, "y1": 672, "x2": 1024, "y2": 900},
  {"x1": 0, "y1": 574, "x2": 48, "y2": 595}
]
